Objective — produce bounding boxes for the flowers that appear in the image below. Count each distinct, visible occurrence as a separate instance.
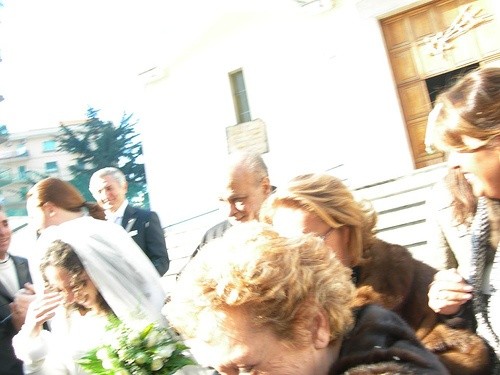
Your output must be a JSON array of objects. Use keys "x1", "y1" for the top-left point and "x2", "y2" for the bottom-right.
[{"x1": 79, "y1": 310, "x2": 196, "y2": 375}]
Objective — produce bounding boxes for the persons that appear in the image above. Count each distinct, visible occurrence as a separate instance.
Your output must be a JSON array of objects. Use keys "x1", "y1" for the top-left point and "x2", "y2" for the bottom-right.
[
  {"x1": 259, "y1": 173, "x2": 494, "y2": 375},
  {"x1": 0, "y1": 166, "x2": 169, "y2": 375},
  {"x1": 174, "y1": 218, "x2": 448, "y2": 375},
  {"x1": 177, "y1": 148, "x2": 278, "y2": 280},
  {"x1": 426, "y1": 65, "x2": 500, "y2": 362}
]
